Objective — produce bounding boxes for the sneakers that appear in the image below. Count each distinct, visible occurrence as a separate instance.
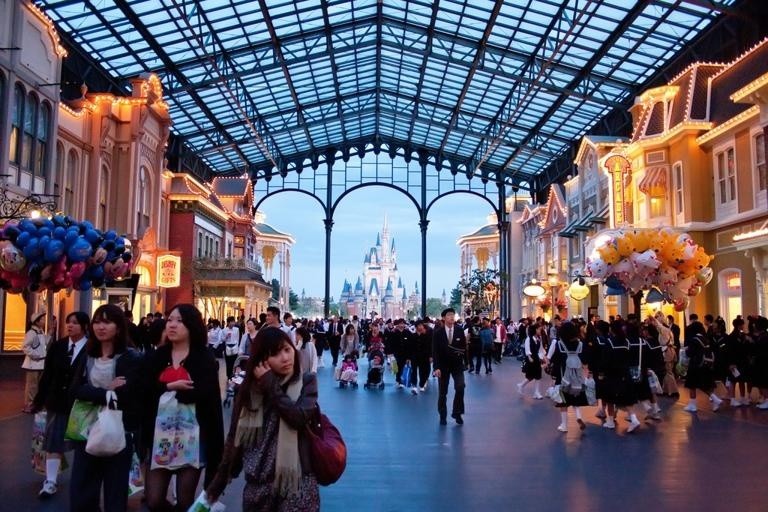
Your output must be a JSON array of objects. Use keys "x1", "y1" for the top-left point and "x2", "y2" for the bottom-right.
[
  {"x1": 440, "y1": 413, "x2": 463, "y2": 424},
  {"x1": 397, "y1": 383, "x2": 425, "y2": 395},
  {"x1": 467, "y1": 367, "x2": 492, "y2": 375},
  {"x1": 558, "y1": 419, "x2": 585, "y2": 432},
  {"x1": 39, "y1": 480, "x2": 57, "y2": 496},
  {"x1": 596, "y1": 409, "x2": 660, "y2": 434},
  {"x1": 518, "y1": 384, "x2": 542, "y2": 399},
  {"x1": 684, "y1": 398, "x2": 768, "y2": 413}
]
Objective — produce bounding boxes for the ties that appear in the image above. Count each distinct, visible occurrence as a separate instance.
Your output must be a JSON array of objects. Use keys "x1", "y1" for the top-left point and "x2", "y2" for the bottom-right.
[{"x1": 69, "y1": 344, "x2": 75, "y2": 364}]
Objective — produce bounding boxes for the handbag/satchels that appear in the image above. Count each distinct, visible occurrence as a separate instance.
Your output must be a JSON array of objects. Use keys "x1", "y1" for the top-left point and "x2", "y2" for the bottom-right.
[
  {"x1": 306, "y1": 402, "x2": 346, "y2": 486},
  {"x1": 648, "y1": 368, "x2": 664, "y2": 395},
  {"x1": 64, "y1": 390, "x2": 126, "y2": 456},
  {"x1": 32, "y1": 413, "x2": 68, "y2": 474},
  {"x1": 630, "y1": 367, "x2": 642, "y2": 382},
  {"x1": 151, "y1": 391, "x2": 200, "y2": 471},
  {"x1": 401, "y1": 362, "x2": 412, "y2": 386}
]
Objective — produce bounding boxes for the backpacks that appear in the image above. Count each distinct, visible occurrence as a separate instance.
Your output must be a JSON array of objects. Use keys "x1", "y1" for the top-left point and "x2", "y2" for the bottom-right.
[{"x1": 561, "y1": 341, "x2": 584, "y2": 396}]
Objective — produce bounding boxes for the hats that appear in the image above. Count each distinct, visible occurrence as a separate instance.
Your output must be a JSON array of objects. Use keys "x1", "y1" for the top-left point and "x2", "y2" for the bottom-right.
[
  {"x1": 31, "y1": 312, "x2": 46, "y2": 323},
  {"x1": 441, "y1": 309, "x2": 455, "y2": 316}
]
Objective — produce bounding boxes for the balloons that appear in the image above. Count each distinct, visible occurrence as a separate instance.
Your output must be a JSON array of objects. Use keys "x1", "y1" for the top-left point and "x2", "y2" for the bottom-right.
[
  {"x1": 584, "y1": 225, "x2": 713, "y2": 311},
  {"x1": 0, "y1": 214, "x2": 135, "y2": 294}
]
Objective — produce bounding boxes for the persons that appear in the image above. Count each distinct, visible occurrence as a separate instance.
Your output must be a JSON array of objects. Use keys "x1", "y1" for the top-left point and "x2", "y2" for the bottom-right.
[
  {"x1": 67, "y1": 304, "x2": 146, "y2": 512},
  {"x1": 517, "y1": 311, "x2": 681, "y2": 433},
  {"x1": 206, "y1": 307, "x2": 335, "y2": 390},
  {"x1": 21, "y1": 312, "x2": 51, "y2": 413},
  {"x1": 138, "y1": 303, "x2": 224, "y2": 512},
  {"x1": 124, "y1": 311, "x2": 170, "y2": 352},
  {"x1": 335, "y1": 318, "x2": 434, "y2": 394},
  {"x1": 432, "y1": 309, "x2": 466, "y2": 424},
  {"x1": 684, "y1": 313, "x2": 767, "y2": 411},
  {"x1": 21, "y1": 311, "x2": 90, "y2": 498},
  {"x1": 308, "y1": 314, "x2": 517, "y2": 375},
  {"x1": 206, "y1": 327, "x2": 320, "y2": 512}
]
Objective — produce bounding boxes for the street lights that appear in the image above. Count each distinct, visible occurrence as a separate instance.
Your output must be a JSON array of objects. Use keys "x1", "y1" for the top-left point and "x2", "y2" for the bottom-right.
[{"x1": 520, "y1": 277, "x2": 570, "y2": 319}]
